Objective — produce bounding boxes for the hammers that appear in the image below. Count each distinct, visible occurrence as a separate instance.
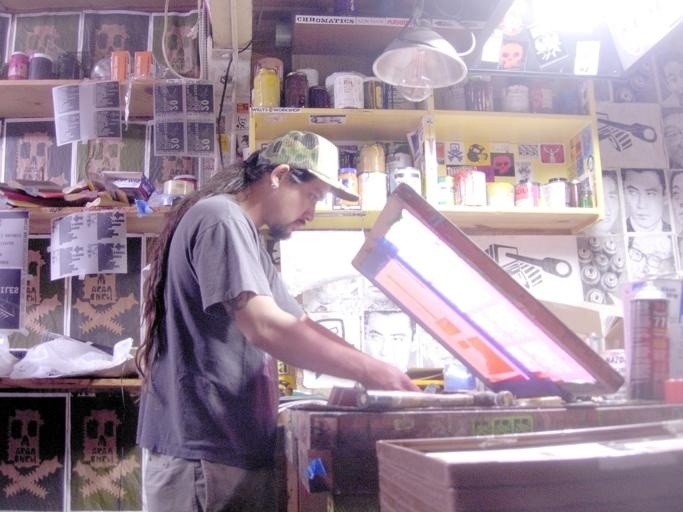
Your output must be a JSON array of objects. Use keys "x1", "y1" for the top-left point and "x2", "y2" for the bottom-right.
[{"x1": 372, "y1": 391, "x2": 493, "y2": 405}]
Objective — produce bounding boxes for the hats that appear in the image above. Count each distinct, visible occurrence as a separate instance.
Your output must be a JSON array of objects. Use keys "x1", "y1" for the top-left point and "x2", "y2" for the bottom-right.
[{"x1": 257, "y1": 130, "x2": 359, "y2": 201}]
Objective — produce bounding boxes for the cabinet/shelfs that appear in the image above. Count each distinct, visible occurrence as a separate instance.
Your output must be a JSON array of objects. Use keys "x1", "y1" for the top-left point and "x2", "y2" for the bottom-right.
[
  {"x1": 248, "y1": 15, "x2": 607, "y2": 232},
  {"x1": 0, "y1": 76, "x2": 168, "y2": 391}
]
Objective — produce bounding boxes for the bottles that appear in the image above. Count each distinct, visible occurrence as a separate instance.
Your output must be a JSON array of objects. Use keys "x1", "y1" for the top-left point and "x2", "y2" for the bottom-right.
[
  {"x1": 7, "y1": 51, "x2": 29, "y2": 80},
  {"x1": 252, "y1": 66, "x2": 281, "y2": 107},
  {"x1": 27, "y1": 52, "x2": 54, "y2": 79},
  {"x1": 309, "y1": 85, "x2": 330, "y2": 108},
  {"x1": 283, "y1": 71, "x2": 310, "y2": 107},
  {"x1": 439, "y1": 169, "x2": 573, "y2": 211},
  {"x1": 333, "y1": 166, "x2": 423, "y2": 213},
  {"x1": 628, "y1": 279, "x2": 671, "y2": 400}
]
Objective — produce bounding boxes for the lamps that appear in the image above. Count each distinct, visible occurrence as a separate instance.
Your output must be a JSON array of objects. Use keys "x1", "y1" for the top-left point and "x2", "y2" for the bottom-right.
[
  {"x1": 631, "y1": 270, "x2": 683, "y2": 303},
  {"x1": 373, "y1": 1, "x2": 477, "y2": 104}
]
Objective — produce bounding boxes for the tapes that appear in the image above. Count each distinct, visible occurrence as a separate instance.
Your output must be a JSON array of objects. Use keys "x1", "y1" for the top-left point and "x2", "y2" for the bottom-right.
[{"x1": 163, "y1": 181, "x2": 194, "y2": 195}]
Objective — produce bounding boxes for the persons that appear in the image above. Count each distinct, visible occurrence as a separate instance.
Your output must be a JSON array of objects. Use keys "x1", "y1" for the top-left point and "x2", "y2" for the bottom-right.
[
  {"x1": 130, "y1": 127, "x2": 421, "y2": 512},
  {"x1": 662, "y1": 109, "x2": 681, "y2": 167},
  {"x1": 589, "y1": 168, "x2": 621, "y2": 228},
  {"x1": 669, "y1": 170, "x2": 681, "y2": 234},
  {"x1": 658, "y1": 52, "x2": 682, "y2": 107},
  {"x1": 619, "y1": 167, "x2": 670, "y2": 234},
  {"x1": 626, "y1": 238, "x2": 674, "y2": 287},
  {"x1": 362, "y1": 297, "x2": 417, "y2": 376}
]
